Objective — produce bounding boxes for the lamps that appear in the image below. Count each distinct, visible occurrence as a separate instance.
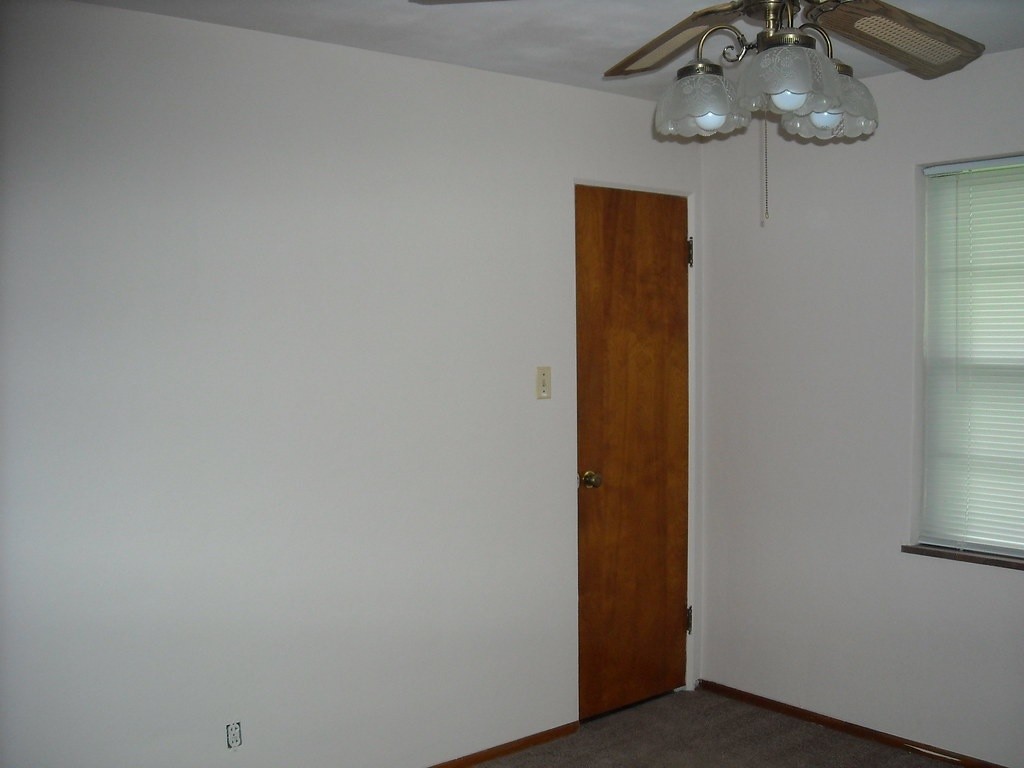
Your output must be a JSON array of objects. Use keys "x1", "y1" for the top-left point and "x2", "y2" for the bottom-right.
[{"x1": 653, "y1": 0, "x2": 879, "y2": 141}]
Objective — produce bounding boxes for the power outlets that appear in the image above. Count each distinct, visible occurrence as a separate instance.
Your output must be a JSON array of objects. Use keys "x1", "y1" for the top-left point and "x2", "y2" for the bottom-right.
[{"x1": 225, "y1": 722, "x2": 243, "y2": 750}]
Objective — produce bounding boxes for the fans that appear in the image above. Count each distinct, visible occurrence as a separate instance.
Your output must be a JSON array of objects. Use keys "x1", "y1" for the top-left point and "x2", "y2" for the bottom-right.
[{"x1": 602, "y1": 0, "x2": 986, "y2": 80}]
